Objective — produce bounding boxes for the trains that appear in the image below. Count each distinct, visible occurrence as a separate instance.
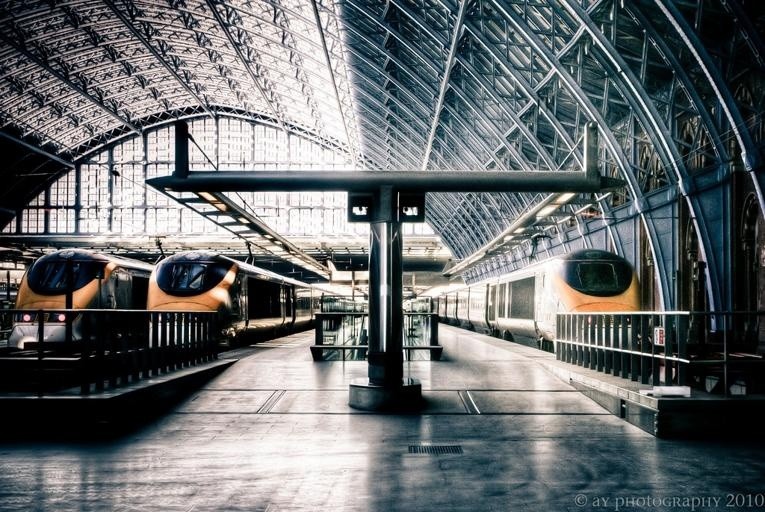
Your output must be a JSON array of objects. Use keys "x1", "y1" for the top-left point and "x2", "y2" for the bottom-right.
[
  {"x1": 404, "y1": 250, "x2": 643, "y2": 353},
  {"x1": 146, "y1": 250, "x2": 369, "y2": 350},
  {"x1": 7, "y1": 248, "x2": 153, "y2": 348}
]
